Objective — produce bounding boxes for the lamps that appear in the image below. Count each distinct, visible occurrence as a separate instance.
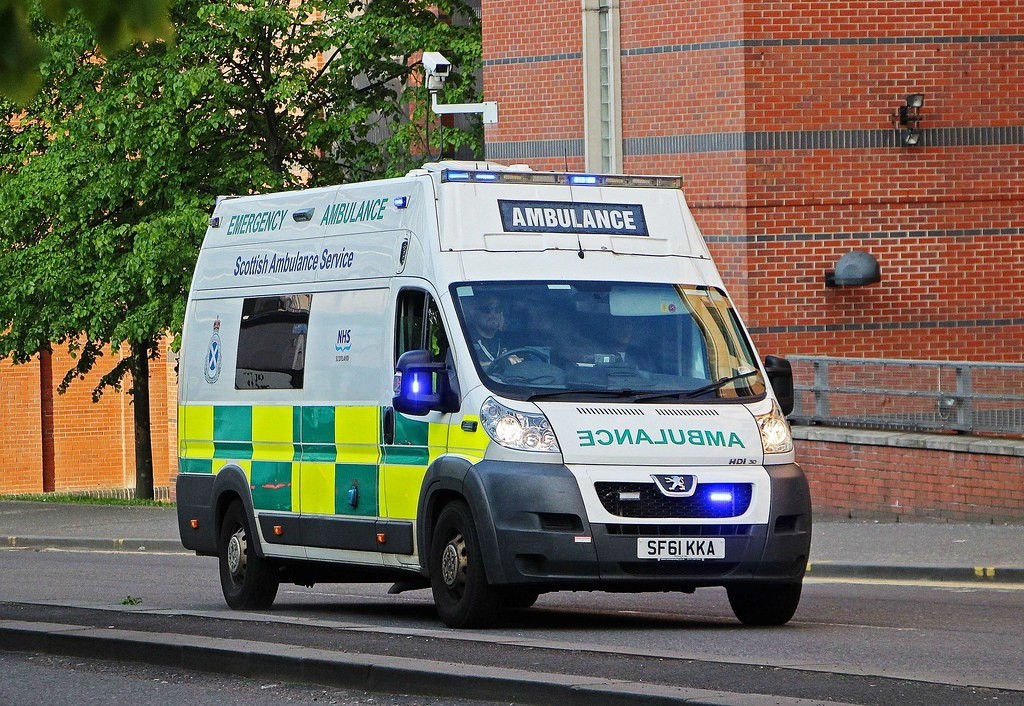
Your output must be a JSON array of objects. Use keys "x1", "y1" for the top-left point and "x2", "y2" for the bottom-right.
[
  {"x1": 900, "y1": 117, "x2": 922, "y2": 147},
  {"x1": 899, "y1": 94, "x2": 924, "y2": 117}
]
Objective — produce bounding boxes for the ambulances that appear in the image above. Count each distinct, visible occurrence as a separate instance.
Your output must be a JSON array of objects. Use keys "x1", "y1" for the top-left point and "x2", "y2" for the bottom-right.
[{"x1": 175, "y1": 160, "x2": 813, "y2": 629}]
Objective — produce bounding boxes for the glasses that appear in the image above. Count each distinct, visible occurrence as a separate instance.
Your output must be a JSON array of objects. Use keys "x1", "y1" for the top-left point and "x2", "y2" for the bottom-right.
[{"x1": 477, "y1": 305, "x2": 502, "y2": 314}]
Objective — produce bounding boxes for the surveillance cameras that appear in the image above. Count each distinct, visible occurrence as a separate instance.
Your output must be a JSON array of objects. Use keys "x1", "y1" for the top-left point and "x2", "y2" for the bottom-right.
[{"x1": 422, "y1": 52, "x2": 452, "y2": 77}]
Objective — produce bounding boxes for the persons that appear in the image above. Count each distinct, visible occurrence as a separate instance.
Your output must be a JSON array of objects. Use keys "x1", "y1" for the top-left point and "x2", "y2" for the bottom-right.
[
  {"x1": 590, "y1": 318, "x2": 650, "y2": 371},
  {"x1": 443, "y1": 291, "x2": 526, "y2": 409}
]
